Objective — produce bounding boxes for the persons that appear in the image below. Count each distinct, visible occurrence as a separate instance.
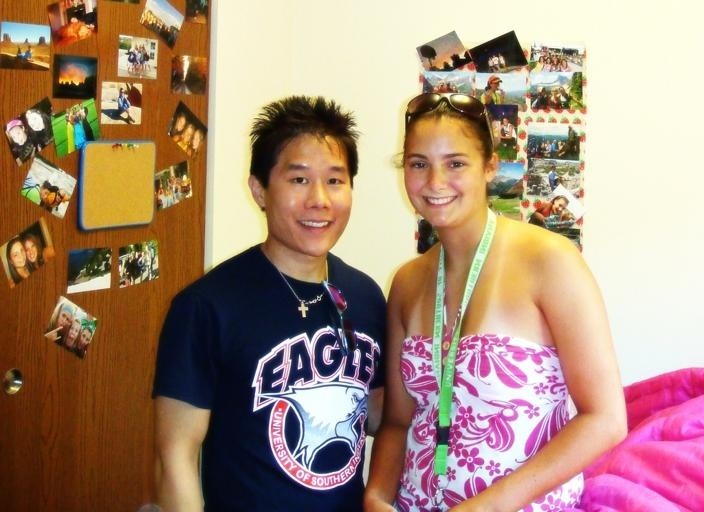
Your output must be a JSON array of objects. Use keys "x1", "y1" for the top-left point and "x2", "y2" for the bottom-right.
[
  {"x1": 417, "y1": 30, "x2": 590, "y2": 251},
  {"x1": 358, "y1": 89, "x2": 631, "y2": 510},
  {"x1": 148, "y1": 95, "x2": 394, "y2": 512}
]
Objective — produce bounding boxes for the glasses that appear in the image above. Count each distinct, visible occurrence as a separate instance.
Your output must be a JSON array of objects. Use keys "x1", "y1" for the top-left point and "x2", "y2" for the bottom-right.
[
  {"x1": 321, "y1": 278, "x2": 357, "y2": 356},
  {"x1": 405, "y1": 93, "x2": 496, "y2": 154}
]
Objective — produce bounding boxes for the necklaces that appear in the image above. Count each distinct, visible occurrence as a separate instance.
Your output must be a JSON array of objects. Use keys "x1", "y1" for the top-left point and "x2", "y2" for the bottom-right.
[{"x1": 266, "y1": 254, "x2": 335, "y2": 321}]
